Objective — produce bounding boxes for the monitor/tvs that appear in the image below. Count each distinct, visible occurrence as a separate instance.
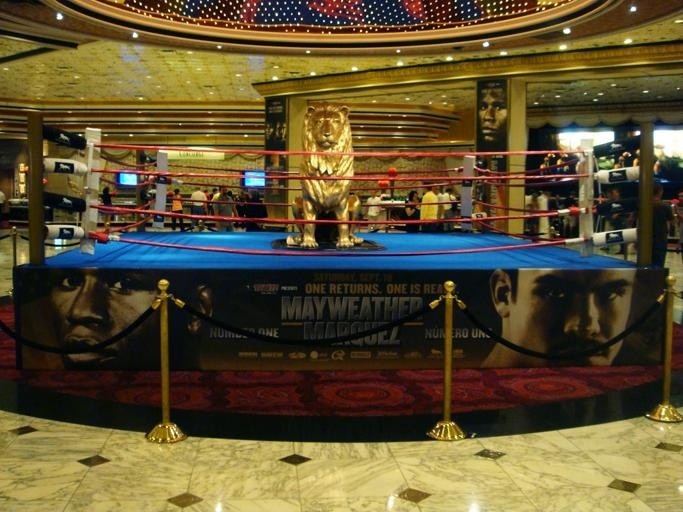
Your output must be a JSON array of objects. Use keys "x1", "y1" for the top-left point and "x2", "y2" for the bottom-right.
[
  {"x1": 117, "y1": 171, "x2": 138, "y2": 186},
  {"x1": 241, "y1": 169, "x2": 266, "y2": 189}
]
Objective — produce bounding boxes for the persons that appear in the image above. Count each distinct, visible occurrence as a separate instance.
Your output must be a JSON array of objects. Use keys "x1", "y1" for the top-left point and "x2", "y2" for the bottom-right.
[
  {"x1": 528, "y1": 198, "x2": 539, "y2": 235},
  {"x1": 550, "y1": 183, "x2": 683, "y2": 268},
  {"x1": 102, "y1": 186, "x2": 115, "y2": 221},
  {"x1": 275, "y1": 121, "x2": 286, "y2": 140},
  {"x1": 49, "y1": 268, "x2": 214, "y2": 373},
  {"x1": 140, "y1": 184, "x2": 268, "y2": 232},
  {"x1": 477, "y1": 88, "x2": 508, "y2": 145},
  {"x1": 348, "y1": 183, "x2": 459, "y2": 233},
  {"x1": 480, "y1": 268, "x2": 637, "y2": 368},
  {"x1": 472, "y1": 200, "x2": 481, "y2": 213}
]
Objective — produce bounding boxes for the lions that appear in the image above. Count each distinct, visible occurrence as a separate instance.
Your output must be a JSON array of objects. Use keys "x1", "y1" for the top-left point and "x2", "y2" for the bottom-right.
[{"x1": 292, "y1": 101, "x2": 366, "y2": 250}]
